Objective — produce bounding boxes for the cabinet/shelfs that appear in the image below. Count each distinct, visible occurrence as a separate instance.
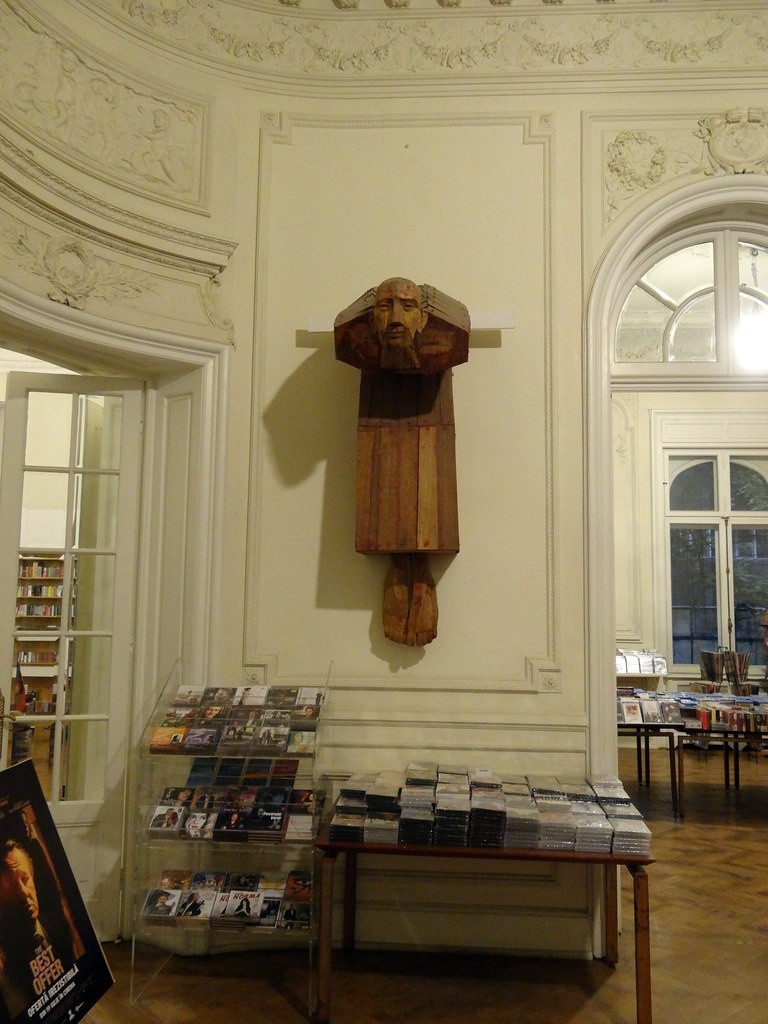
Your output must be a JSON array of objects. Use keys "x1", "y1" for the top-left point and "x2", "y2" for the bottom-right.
[
  {"x1": 130, "y1": 655, "x2": 338, "y2": 1016},
  {"x1": 689, "y1": 679, "x2": 763, "y2": 765},
  {"x1": 6, "y1": 554, "x2": 79, "y2": 677}
]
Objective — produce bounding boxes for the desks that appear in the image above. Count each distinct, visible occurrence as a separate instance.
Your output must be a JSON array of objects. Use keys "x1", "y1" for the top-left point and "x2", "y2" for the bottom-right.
[
  {"x1": 616, "y1": 720, "x2": 768, "y2": 818},
  {"x1": 315, "y1": 787, "x2": 657, "y2": 1024}
]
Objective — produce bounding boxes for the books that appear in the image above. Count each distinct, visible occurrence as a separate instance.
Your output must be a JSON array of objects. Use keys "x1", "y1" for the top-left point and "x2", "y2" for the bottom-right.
[
  {"x1": 11, "y1": 555, "x2": 78, "y2": 765},
  {"x1": 616, "y1": 648, "x2": 768, "y2": 740},
  {"x1": 141, "y1": 684, "x2": 652, "y2": 936}
]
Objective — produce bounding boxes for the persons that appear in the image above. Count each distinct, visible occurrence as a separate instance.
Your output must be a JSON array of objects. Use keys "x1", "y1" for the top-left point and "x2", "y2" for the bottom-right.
[
  {"x1": 177, "y1": 892, "x2": 202, "y2": 916},
  {"x1": 200, "y1": 704, "x2": 220, "y2": 723},
  {"x1": 222, "y1": 810, "x2": 240, "y2": 832},
  {"x1": 152, "y1": 895, "x2": 170, "y2": 916},
  {"x1": 0, "y1": 838, "x2": 70, "y2": 989},
  {"x1": 184, "y1": 813, "x2": 207, "y2": 838},
  {"x1": 209, "y1": 685, "x2": 232, "y2": 704},
  {"x1": 335, "y1": 278, "x2": 472, "y2": 646},
  {"x1": 302, "y1": 705, "x2": 319, "y2": 720},
  {"x1": 234, "y1": 710, "x2": 259, "y2": 727},
  {"x1": 166, "y1": 788, "x2": 192, "y2": 806},
  {"x1": 234, "y1": 898, "x2": 250, "y2": 916},
  {"x1": 289, "y1": 729, "x2": 309, "y2": 753},
  {"x1": 151, "y1": 806, "x2": 179, "y2": 829}
]
